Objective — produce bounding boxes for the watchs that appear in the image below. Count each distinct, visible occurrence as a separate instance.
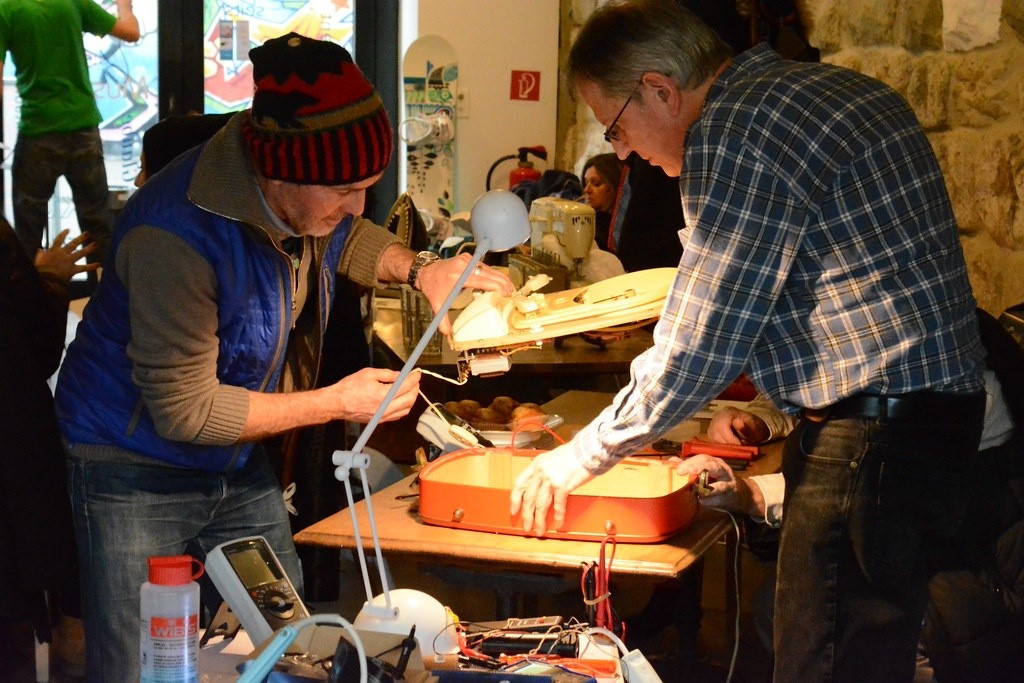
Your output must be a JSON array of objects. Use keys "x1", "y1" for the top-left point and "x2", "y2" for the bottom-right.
[{"x1": 408, "y1": 251, "x2": 441, "y2": 291}]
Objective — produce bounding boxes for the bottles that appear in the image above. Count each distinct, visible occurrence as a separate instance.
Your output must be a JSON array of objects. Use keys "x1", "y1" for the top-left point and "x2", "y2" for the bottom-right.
[{"x1": 139, "y1": 554, "x2": 204, "y2": 683}]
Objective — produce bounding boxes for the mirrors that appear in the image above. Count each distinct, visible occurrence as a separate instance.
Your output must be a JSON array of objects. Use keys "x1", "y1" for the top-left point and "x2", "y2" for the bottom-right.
[{"x1": 402, "y1": 32, "x2": 459, "y2": 253}]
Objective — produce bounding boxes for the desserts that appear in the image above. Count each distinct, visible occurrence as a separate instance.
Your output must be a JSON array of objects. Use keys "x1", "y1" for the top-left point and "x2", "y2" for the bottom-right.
[{"x1": 442, "y1": 395, "x2": 545, "y2": 433}]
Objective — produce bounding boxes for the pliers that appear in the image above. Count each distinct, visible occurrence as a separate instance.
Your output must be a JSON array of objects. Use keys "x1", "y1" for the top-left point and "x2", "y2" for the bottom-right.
[
  {"x1": 652, "y1": 439, "x2": 759, "y2": 460},
  {"x1": 681, "y1": 454, "x2": 748, "y2": 470}
]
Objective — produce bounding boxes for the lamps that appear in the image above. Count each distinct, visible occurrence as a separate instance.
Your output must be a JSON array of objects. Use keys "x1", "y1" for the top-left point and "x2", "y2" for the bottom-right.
[{"x1": 331, "y1": 188, "x2": 534, "y2": 618}]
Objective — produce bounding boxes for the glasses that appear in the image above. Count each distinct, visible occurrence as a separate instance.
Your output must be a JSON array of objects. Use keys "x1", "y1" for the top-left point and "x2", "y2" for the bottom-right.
[{"x1": 604, "y1": 68, "x2": 671, "y2": 146}]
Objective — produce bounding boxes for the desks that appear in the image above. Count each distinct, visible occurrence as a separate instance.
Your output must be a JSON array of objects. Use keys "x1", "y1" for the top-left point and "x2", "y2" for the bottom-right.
[
  {"x1": 365, "y1": 295, "x2": 661, "y2": 387},
  {"x1": 288, "y1": 388, "x2": 807, "y2": 683},
  {"x1": 182, "y1": 621, "x2": 627, "y2": 683}
]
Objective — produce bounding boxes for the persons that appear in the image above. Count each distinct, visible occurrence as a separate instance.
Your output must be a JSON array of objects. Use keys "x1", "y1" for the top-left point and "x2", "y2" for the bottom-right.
[
  {"x1": 0, "y1": 0, "x2": 718, "y2": 683},
  {"x1": 507, "y1": 0, "x2": 1024, "y2": 683}
]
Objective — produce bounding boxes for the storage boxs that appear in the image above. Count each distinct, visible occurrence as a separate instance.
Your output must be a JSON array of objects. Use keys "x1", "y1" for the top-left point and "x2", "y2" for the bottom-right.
[
  {"x1": 504, "y1": 248, "x2": 571, "y2": 295},
  {"x1": 403, "y1": 441, "x2": 710, "y2": 545}
]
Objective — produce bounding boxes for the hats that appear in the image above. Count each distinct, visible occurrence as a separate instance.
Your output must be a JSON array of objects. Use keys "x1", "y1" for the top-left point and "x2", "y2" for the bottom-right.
[{"x1": 248, "y1": 32, "x2": 392, "y2": 186}]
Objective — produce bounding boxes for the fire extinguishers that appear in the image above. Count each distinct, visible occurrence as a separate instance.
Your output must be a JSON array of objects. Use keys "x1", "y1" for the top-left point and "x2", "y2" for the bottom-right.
[{"x1": 486, "y1": 147, "x2": 548, "y2": 191}]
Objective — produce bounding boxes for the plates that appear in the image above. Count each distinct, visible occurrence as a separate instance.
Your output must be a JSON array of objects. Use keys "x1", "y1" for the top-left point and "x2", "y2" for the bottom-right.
[{"x1": 478, "y1": 413, "x2": 565, "y2": 449}]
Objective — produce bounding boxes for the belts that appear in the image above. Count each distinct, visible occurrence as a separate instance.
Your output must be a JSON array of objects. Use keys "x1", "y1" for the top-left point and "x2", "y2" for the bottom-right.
[{"x1": 829, "y1": 388, "x2": 988, "y2": 418}]
